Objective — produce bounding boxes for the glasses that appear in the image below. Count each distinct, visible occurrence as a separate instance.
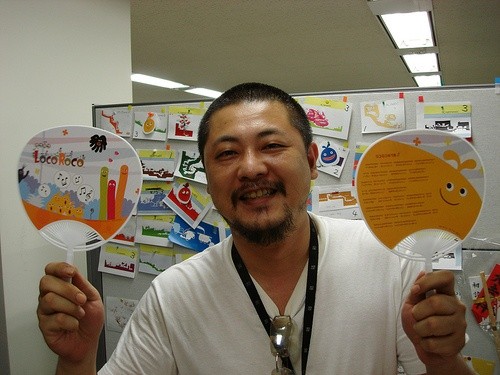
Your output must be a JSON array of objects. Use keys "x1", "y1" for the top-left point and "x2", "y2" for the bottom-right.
[{"x1": 270, "y1": 315, "x2": 300, "y2": 375}]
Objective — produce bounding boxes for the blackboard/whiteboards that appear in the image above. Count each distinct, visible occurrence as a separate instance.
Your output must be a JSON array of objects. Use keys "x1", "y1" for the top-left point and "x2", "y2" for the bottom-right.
[{"x1": 86, "y1": 82, "x2": 500, "y2": 375}]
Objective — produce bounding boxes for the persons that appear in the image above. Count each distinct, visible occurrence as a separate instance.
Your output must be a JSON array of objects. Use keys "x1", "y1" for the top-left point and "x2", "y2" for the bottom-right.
[{"x1": 37, "y1": 82, "x2": 468, "y2": 375}]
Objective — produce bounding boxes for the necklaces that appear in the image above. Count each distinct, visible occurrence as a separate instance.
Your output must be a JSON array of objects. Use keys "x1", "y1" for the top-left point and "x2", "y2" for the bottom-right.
[{"x1": 231, "y1": 211, "x2": 319, "y2": 375}]
